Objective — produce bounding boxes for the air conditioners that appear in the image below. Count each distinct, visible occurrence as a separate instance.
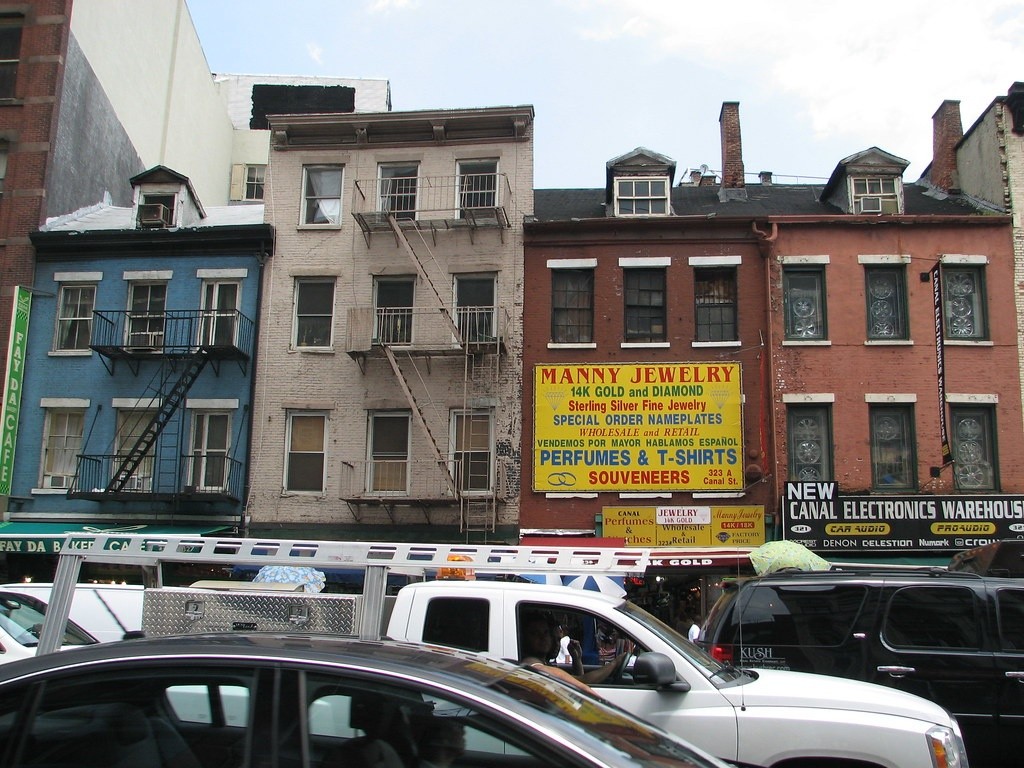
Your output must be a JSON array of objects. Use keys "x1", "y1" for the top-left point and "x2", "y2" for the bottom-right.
[
  {"x1": 50, "y1": 475, "x2": 70, "y2": 488},
  {"x1": 119, "y1": 475, "x2": 138, "y2": 489},
  {"x1": 138, "y1": 203, "x2": 170, "y2": 226},
  {"x1": 128, "y1": 333, "x2": 154, "y2": 347}
]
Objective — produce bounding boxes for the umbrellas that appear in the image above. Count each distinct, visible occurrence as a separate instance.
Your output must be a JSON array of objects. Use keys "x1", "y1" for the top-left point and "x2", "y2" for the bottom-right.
[
  {"x1": 745, "y1": 540, "x2": 833, "y2": 577},
  {"x1": 514, "y1": 573, "x2": 628, "y2": 599},
  {"x1": 251, "y1": 565, "x2": 326, "y2": 593}
]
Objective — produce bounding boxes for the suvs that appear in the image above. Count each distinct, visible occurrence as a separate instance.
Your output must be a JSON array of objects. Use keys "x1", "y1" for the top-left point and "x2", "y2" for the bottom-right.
[{"x1": 693, "y1": 568, "x2": 1024, "y2": 768}]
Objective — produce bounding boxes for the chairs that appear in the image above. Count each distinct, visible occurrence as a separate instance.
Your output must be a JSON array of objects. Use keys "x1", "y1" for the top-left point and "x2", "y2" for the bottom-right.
[{"x1": 345, "y1": 689, "x2": 407, "y2": 768}]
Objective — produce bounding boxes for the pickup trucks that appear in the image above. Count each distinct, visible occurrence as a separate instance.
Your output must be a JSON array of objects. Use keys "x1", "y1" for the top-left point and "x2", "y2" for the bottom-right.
[{"x1": 143, "y1": 581, "x2": 968, "y2": 767}]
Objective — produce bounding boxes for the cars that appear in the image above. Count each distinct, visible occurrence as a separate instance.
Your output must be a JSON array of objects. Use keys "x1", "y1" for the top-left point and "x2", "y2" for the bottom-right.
[
  {"x1": 0, "y1": 635, "x2": 740, "y2": 768},
  {"x1": 0, "y1": 585, "x2": 103, "y2": 665}
]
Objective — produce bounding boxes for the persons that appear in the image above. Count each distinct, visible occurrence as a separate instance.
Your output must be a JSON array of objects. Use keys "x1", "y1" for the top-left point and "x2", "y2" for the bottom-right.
[
  {"x1": 688, "y1": 614, "x2": 702, "y2": 647},
  {"x1": 519, "y1": 610, "x2": 636, "y2": 684}
]
